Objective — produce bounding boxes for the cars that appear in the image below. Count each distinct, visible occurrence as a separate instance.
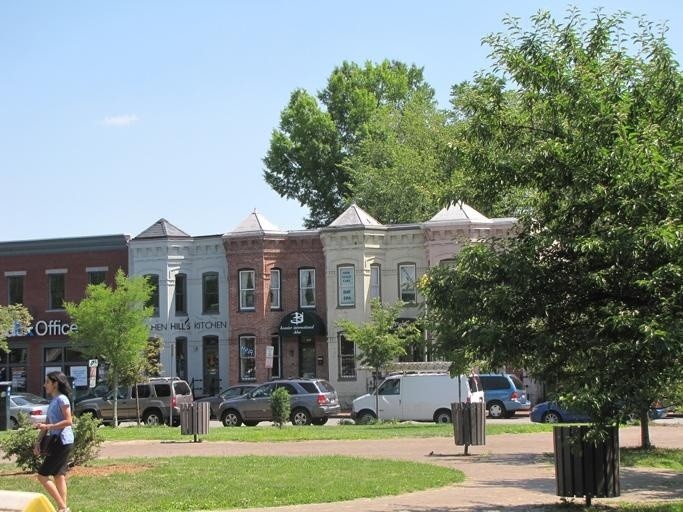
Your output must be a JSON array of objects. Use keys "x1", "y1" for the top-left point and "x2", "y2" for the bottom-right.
[
  {"x1": 528, "y1": 398, "x2": 667, "y2": 424},
  {"x1": 195, "y1": 385, "x2": 260, "y2": 420},
  {"x1": 1, "y1": 392, "x2": 53, "y2": 430}
]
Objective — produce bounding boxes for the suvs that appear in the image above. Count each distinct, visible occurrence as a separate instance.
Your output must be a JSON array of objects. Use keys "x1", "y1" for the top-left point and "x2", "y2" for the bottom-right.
[
  {"x1": 72, "y1": 377, "x2": 194, "y2": 428},
  {"x1": 467, "y1": 373, "x2": 532, "y2": 419},
  {"x1": 218, "y1": 377, "x2": 341, "y2": 427}
]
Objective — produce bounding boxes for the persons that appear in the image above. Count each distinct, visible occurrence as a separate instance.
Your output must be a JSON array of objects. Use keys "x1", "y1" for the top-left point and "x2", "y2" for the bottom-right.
[{"x1": 35, "y1": 371, "x2": 74, "y2": 512}]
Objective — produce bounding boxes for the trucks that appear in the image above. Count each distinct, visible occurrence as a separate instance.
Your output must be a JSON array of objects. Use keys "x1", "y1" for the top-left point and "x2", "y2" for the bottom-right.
[{"x1": 350, "y1": 370, "x2": 486, "y2": 425}]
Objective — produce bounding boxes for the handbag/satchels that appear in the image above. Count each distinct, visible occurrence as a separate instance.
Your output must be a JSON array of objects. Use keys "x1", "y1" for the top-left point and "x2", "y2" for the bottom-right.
[{"x1": 39, "y1": 434, "x2": 58, "y2": 457}]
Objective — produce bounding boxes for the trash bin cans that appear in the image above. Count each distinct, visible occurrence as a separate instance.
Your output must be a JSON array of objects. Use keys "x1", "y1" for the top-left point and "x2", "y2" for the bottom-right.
[
  {"x1": 451, "y1": 402, "x2": 486, "y2": 454},
  {"x1": 180, "y1": 401, "x2": 209, "y2": 442},
  {"x1": 553, "y1": 425, "x2": 621, "y2": 507}
]
